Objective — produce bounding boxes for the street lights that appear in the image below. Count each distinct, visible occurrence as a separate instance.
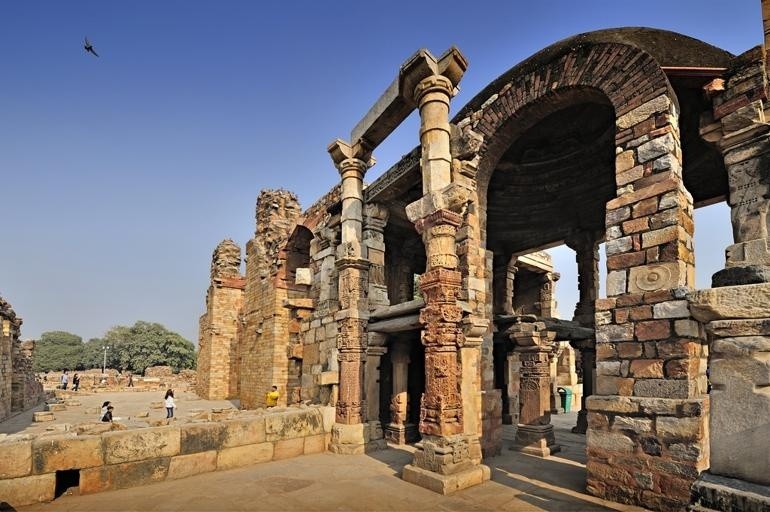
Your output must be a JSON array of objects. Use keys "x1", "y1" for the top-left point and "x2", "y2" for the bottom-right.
[{"x1": 102, "y1": 346, "x2": 109, "y2": 373}]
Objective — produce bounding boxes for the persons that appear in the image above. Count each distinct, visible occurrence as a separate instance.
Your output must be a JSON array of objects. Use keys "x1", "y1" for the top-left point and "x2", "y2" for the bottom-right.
[
  {"x1": 100, "y1": 400, "x2": 111, "y2": 420},
  {"x1": 60, "y1": 369, "x2": 69, "y2": 390},
  {"x1": 165, "y1": 388, "x2": 177, "y2": 419},
  {"x1": 128, "y1": 374, "x2": 134, "y2": 387},
  {"x1": 102, "y1": 404, "x2": 114, "y2": 423},
  {"x1": 127, "y1": 373, "x2": 130, "y2": 387},
  {"x1": 73, "y1": 373, "x2": 83, "y2": 390},
  {"x1": 266, "y1": 385, "x2": 279, "y2": 409}
]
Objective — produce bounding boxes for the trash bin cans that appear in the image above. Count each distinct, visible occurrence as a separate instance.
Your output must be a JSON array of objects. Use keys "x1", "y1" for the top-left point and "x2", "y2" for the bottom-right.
[{"x1": 557, "y1": 387, "x2": 573, "y2": 413}]
